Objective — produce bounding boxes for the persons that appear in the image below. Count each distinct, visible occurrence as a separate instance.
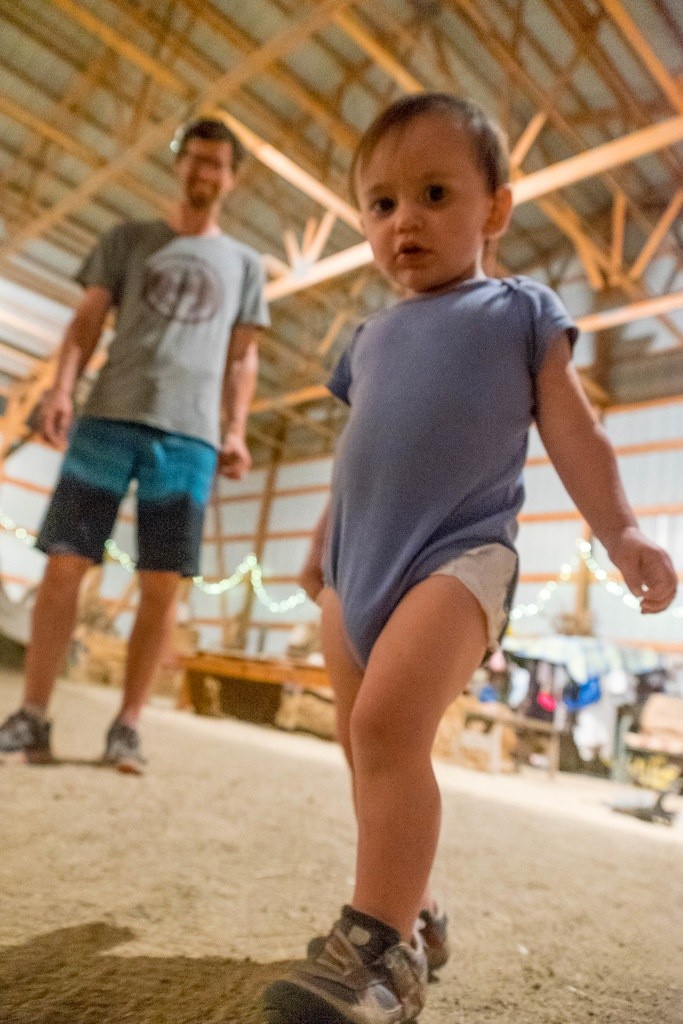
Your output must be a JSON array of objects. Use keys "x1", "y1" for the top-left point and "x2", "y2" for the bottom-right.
[
  {"x1": 0, "y1": 119, "x2": 269, "y2": 772},
  {"x1": 262, "y1": 91, "x2": 679, "y2": 1024}
]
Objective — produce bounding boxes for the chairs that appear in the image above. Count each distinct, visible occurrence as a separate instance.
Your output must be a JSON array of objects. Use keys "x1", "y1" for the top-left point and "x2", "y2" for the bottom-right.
[{"x1": 623, "y1": 692, "x2": 683, "y2": 760}]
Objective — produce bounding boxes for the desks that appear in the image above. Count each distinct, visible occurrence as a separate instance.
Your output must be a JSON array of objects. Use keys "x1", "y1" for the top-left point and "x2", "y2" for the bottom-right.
[{"x1": 164, "y1": 648, "x2": 518, "y2": 774}]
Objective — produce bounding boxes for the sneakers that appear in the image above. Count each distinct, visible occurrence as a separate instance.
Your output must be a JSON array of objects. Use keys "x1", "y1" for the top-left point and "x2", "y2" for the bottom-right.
[
  {"x1": 264, "y1": 901, "x2": 449, "y2": 1024},
  {"x1": 103, "y1": 721, "x2": 148, "y2": 774},
  {"x1": 0, "y1": 709, "x2": 52, "y2": 764}
]
